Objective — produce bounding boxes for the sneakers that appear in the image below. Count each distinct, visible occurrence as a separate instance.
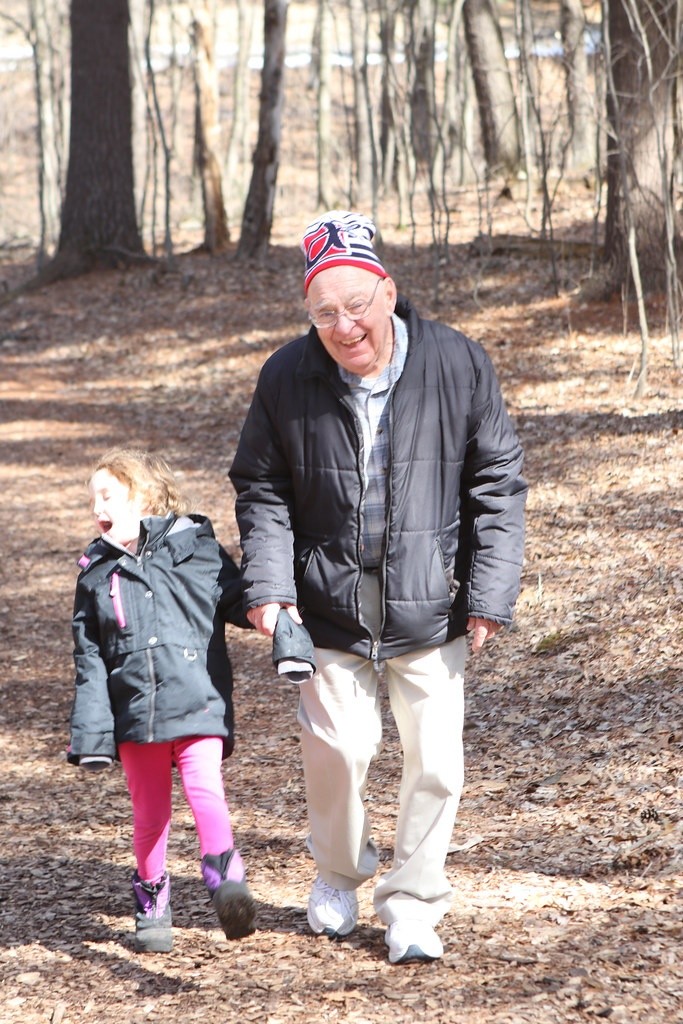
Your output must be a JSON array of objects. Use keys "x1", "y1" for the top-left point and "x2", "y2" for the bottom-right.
[
  {"x1": 384, "y1": 920, "x2": 444, "y2": 964},
  {"x1": 307, "y1": 876, "x2": 359, "y2": 938}
]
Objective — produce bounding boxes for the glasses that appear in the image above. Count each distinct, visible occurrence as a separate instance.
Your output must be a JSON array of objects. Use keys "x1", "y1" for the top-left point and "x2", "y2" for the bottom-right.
[{"x1": 308, "y1": 278, "x2": 382, "y2": 329}]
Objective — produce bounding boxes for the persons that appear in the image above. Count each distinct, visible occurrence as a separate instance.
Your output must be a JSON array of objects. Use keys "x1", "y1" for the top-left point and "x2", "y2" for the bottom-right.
[
  {"x1": 227, "y1": 209, "x2": 529, "y2": 963},
  {"x1": 67, "y1": 447, "x2": 316, "y2": 953}
]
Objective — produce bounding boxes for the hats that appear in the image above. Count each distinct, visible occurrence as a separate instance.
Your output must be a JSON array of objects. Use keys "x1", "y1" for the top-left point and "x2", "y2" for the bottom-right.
[{"x1": 300, "y1": 211, "x2": 391, "y2": 298}]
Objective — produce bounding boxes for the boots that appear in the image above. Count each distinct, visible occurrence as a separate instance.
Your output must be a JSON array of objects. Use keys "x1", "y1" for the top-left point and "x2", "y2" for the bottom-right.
[
  {"x1": 200, "y1": 849, "x2": 257, "y2": 941},
  {"x1": 131, "y1": 868, "x2": 174, "y2": 952}
]
